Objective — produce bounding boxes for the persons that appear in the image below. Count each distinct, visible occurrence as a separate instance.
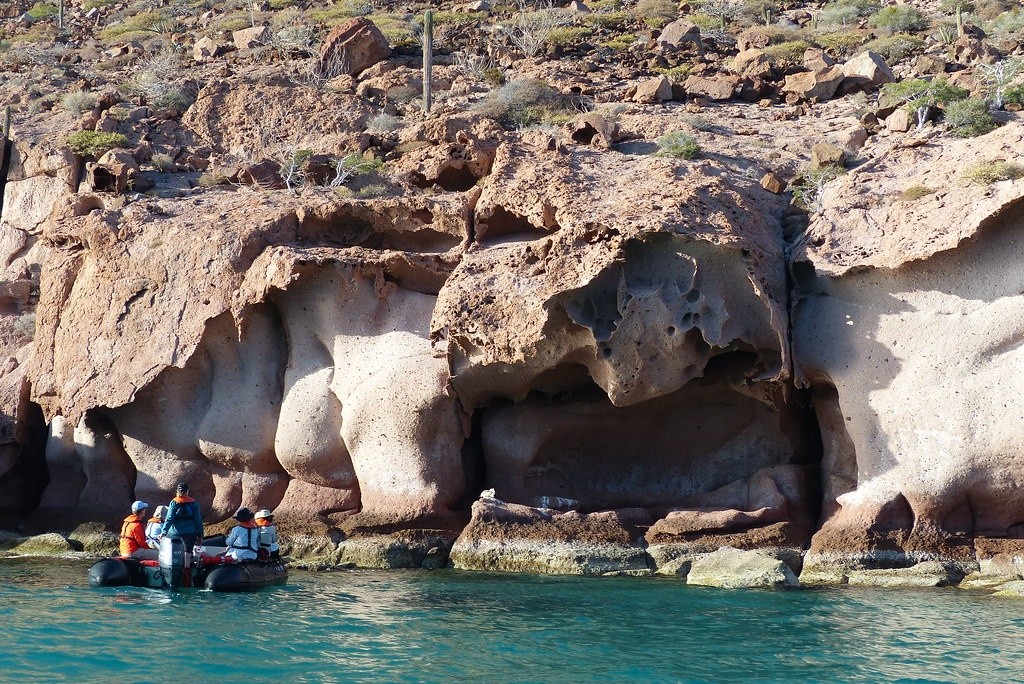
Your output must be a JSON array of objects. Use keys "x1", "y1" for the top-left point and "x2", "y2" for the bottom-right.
[
  {"x1": 119, "y1": 483, "x2": 204, "y2": 568},
  {"x1": 225, "y1": 508, "x2": 279, "y2": 562}
]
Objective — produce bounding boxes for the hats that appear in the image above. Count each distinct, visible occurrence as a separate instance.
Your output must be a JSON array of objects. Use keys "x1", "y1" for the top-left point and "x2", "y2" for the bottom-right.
[
  {"x1": 232, "y1": 509, "x2": 253, "y2": 522},
  {"x1": 255, "y1": 510, "x2": 274, "y2": 519},
  {"x1": 132, "y1": 500, "x2": 149, "y2": 511},
  {"x1": 153, "y1": 505, "x2": 169, "y2": 519}
]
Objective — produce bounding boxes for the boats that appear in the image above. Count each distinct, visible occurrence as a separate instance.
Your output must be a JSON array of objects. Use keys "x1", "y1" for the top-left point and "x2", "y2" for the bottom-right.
[{"x1": 88, "y1": 533, "x2": 290, "y2": 594}]
